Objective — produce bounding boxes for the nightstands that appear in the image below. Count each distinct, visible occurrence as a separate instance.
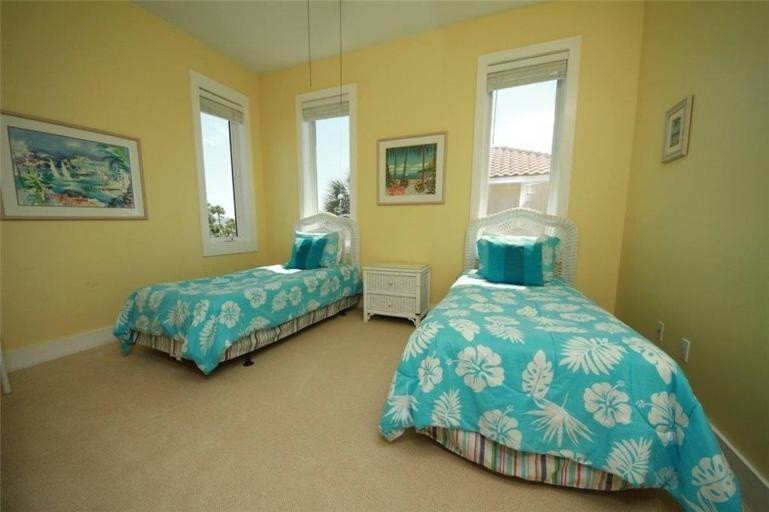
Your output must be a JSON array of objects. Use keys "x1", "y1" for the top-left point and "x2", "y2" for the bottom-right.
[{"x1": 360, "y1": 262, "x2": 431, "y2": 328}]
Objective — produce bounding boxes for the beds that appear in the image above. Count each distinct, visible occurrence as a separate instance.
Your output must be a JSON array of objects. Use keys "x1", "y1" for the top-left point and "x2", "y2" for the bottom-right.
[
  {"x1": 411, "y1": 206, "x2": 685, "y2": 493},
  {"x1": 130, "y1": 211, "x2": 361, "y2": 366}
]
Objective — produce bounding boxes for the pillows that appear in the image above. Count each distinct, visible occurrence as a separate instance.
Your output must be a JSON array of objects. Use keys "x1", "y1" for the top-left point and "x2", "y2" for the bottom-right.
[
  {"x1": 472, "y1": 238, "x2": 562, "y2": 287},
  {"x1": 283, "y1": 232, "x2": 342, "y2": 268}
]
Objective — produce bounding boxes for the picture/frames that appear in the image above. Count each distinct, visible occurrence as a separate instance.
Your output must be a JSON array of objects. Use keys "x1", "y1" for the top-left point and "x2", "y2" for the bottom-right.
[
  {"x1": 0, "y1": 108, "x2": 149, "y2": 221},
  {"x1": 377, "y1": 131, "x2": 447, "y2": 206},
  {"x1": 659, "y1": 94, "x2": 694, "y2": 164}
]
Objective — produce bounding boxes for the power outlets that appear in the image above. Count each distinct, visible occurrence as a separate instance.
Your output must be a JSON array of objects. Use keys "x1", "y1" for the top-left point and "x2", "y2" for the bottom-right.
[
  {"x1": 677, "y1": 338, "x2": 691, "y2": 362},
  {"x1": 655, "y1": 319, "x2": 664, "y2": 342}
]
